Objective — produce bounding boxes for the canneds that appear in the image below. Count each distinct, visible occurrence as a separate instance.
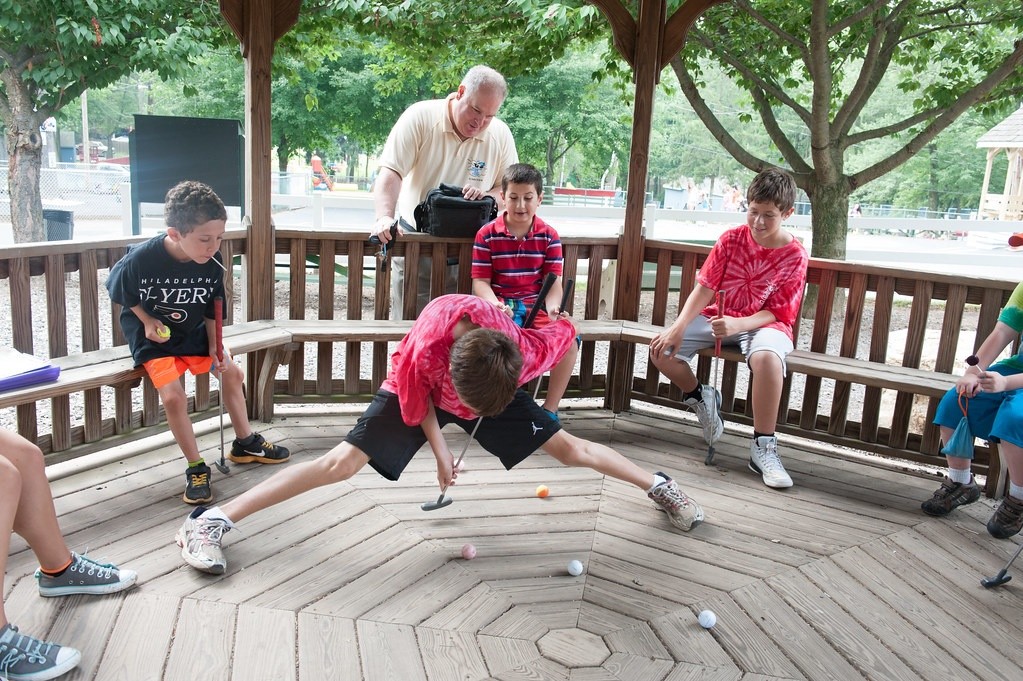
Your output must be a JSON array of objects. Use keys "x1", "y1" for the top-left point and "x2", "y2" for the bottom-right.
[{"x1": 505, "y1": 298, "x2": 523, "y2": 327}]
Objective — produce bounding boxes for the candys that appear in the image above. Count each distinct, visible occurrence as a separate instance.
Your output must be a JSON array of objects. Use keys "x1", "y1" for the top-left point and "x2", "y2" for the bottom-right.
[{"x1": 966, "y1": 355, "x2": 982, "y2": 372}]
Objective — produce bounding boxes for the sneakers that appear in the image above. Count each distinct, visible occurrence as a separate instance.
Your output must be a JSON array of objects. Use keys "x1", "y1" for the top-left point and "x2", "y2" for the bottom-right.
[
  {"x1": 35, "y1": 547, "x2": 138, "y2": 596},
  {"x1": 747, "y1": 436, "x2": 793, "y2": 487},
  {"x1": 183, "y1": 462, "x2": 213, "y2": 504},
  {"x1": 681, "y1": 383, "x2": 724, "y2": 444},
  {"x1": 647, "y1": 471, "x2": 705, "y2": 531},
  {"x1": 0, "y1": 620, "x2": 81, "y2": 681},
  {"x1": 921, "y1": 474, "x2": 980, "y2": 517},
  {"x1": 986, "y1": 488, "x2": 1023, "y2": 539},
  {"x1": 228, "y1": 432, "x2": 291, "y2": 463},
  {"x1": 175, "y1": 507, "x2": 241, "y2": 575}
]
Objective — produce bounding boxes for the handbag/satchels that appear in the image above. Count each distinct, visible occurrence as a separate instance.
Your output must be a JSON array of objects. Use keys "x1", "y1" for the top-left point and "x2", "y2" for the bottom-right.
[
  {"x1": 413, "y1": 182, "x2": 498, "y2": 237},
  {"x1": 940, "y1": 390, "x2": 974, "y2": 459}
]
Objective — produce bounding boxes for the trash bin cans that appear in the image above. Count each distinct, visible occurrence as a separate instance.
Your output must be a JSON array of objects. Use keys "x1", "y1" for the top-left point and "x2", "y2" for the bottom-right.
[{"x1": 42, "y1": 209, "x2": 74, "y2": 241}]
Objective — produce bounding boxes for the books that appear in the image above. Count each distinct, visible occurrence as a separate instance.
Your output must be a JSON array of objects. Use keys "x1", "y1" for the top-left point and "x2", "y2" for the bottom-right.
[{"x1": 0, "y1": 345, "x2": 51, "y2": 381}]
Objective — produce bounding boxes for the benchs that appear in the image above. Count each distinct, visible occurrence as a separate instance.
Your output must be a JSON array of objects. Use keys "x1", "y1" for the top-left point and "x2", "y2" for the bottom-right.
[{"x1": 0, "y1": 318, "x2": 1008, "y2": 500}]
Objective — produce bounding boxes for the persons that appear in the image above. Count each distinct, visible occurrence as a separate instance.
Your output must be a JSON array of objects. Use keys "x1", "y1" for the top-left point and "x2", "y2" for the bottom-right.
[
  {"x1": 613, "y1": 186, "x2": 622, "y2": 207},
  {"x1": 176, "y1": 293, "x2": 704, "y2": 576},
  {"x1": 852, "y1": 201, "x2": 862, "y2": 215},
  {"x1": 649, "y1": 166, "x2": 809, "y2": 488},
  {"x1": 105, "y1": 181, "x2": 290, "y2": 506},
  {"x1": 684, "y1": 180, "x2": 712, "y2": 212},
  {"x1": 0, "y1": 425, "x2": 137, "y2": 681},
  {"x1": 726, "y1": 185, "x2": 745, "y2": 213},
  {"x1": 371, "y1": 64, "x2": 521, "y2": 319},
  {"x1": 918, "y1": 280, "x2": 1022, "y2": 541},
  {"x1": 470, "y1": 164, "x2": 581, "y2": 428}
]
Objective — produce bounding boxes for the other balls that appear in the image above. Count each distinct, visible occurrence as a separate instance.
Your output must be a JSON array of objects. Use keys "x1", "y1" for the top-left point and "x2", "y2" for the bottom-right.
[
  {"x1": 537, "y1": 485, "x2": 549, "y2": 498},
  {"x1": 698, "y1": 610, "x2": 717, "y2": 628},
  {"x1": 567, "y1": 560, "x2": 584, "y2": 576},
  {"x1": 461, "y1": 544, "x2": 476, "y2": 560}
]
